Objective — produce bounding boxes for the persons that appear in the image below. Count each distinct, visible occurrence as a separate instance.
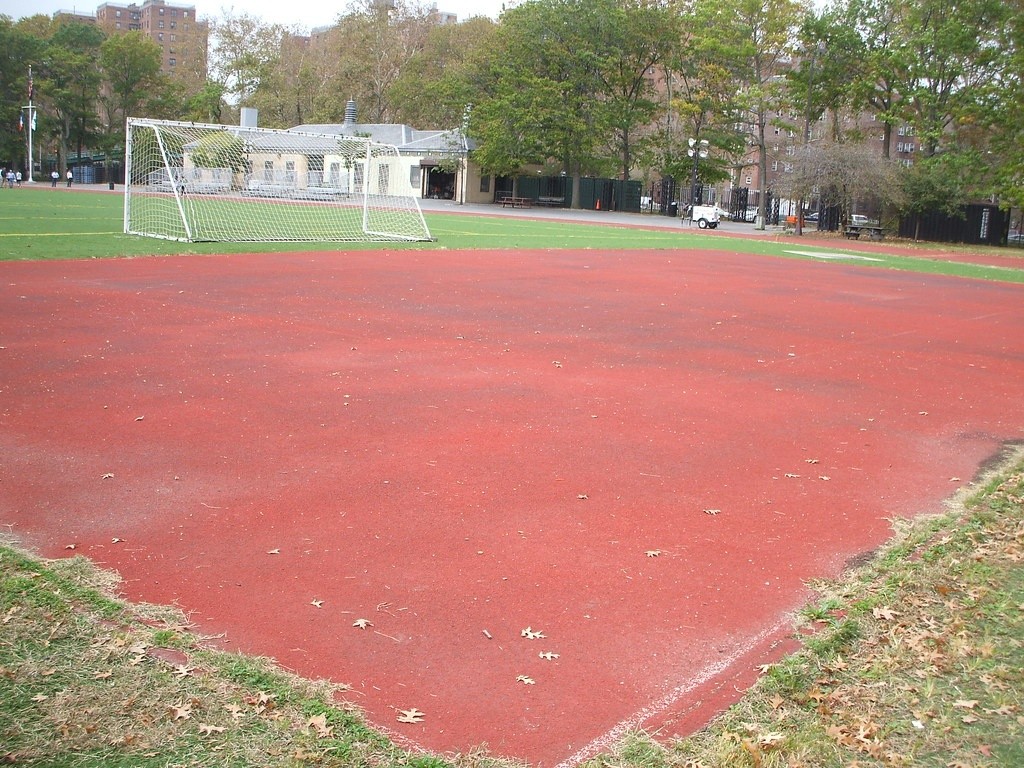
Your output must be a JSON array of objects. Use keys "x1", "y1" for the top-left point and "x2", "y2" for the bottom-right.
[
  {"x1": 1, "y1": 167, "x2": 22, "y2": 189},
  {"x1": 50, "y1": 167, "x2": 61, "y2": 187},
  {"x1": 66, "y1": 169, "x2": 73, "y2": 187}
]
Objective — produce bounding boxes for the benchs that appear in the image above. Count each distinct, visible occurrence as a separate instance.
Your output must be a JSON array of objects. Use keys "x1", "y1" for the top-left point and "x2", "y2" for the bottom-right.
[
  {"x1": 498, "y1": 201, "x2": 535, "y2": 205},
  {"x1": 842, "y1": 231, "x2": 860, "y2": 237},
  {"x1": 866, "y1": 233, "x2": 885, "y2": 236}
]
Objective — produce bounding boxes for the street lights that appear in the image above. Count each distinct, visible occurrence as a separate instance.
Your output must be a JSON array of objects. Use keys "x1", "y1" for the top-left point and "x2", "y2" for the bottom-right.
[{"x1": 688, "y1": 138, "x2": 709, "y2": 219}]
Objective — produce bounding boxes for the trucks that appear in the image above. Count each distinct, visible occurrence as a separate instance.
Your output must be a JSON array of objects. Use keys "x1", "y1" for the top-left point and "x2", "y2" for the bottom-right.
[{"x1": 640, "y1": 196, "x2": 660, "y2": 210}]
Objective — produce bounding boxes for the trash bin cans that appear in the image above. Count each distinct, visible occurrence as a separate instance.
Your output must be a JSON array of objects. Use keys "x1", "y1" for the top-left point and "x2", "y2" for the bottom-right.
[{"x1": 109, "y1": 182, "x2": 114, "y2": 190}]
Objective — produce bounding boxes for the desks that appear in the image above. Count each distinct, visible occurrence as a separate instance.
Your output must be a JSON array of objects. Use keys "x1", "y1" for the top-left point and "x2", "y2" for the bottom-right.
[
  {"x1": 501, "y1": 197, "x2": 534, "y2": 208},
  {"x1": 845, "y1": 225, "x2": 891, "y2": 241}
]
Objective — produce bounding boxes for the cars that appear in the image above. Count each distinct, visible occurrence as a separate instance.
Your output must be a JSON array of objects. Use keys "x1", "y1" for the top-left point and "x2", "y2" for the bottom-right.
[
  {"x1": 734, "y1": 205, "x2": 760, "y2": 223},
  {"x1": 671, "y1": 202, "x2": 686, "y2": 210},
  {"x1": 852, "y1": 215, "x2": 868, "y2": 227},
  {"x1": 804, "y1": 213, "x2": 819, "y2": 221}
]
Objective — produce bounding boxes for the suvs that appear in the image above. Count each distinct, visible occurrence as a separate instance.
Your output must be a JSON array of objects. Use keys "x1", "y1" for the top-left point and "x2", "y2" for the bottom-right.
[{"x1": 703, "y1": 203, "x2": 732, "y2": 219}]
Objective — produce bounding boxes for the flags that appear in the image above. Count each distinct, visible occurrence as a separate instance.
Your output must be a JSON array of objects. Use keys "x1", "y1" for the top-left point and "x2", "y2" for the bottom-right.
[
  {"x1": 17, "y1": 104, "x2": 27, "y2": 133},
  {"x1": 31, "y1": 110, "x2": 37, "y2": 132},
  {"x1": 26, "y1": 76, "x2": 33, "y2": 99}
]
